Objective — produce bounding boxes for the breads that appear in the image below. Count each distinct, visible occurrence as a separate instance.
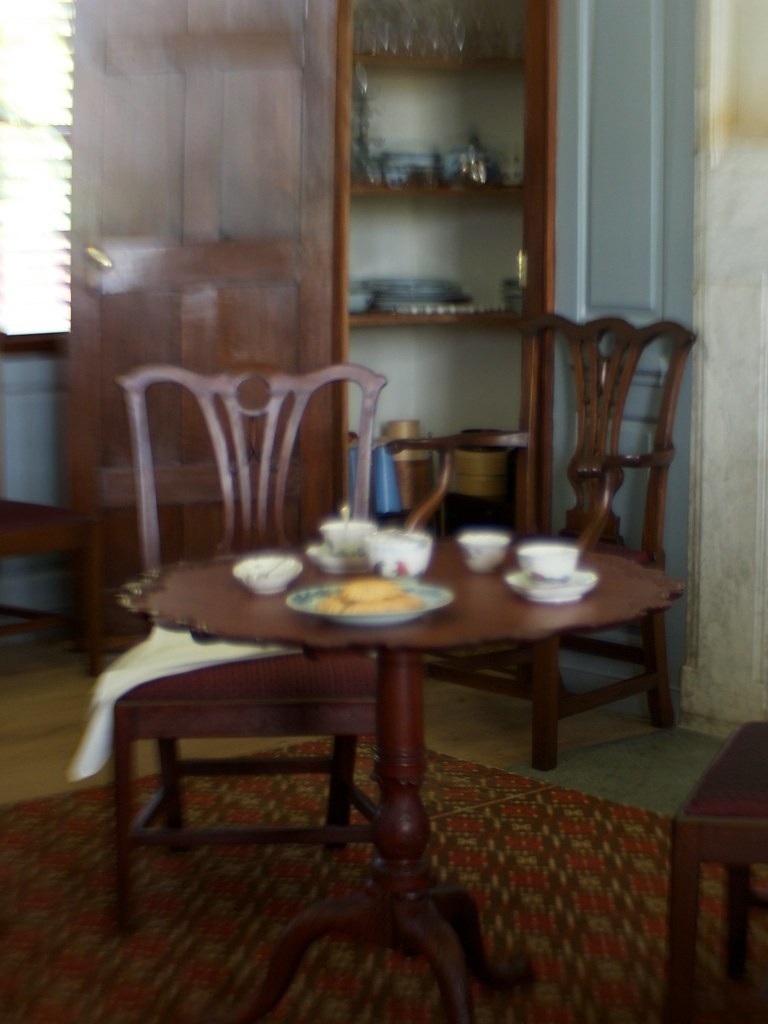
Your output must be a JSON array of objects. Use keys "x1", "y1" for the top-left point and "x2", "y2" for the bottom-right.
[{"x1": 315, "y1": 579, "x2": 423, "y2": 616}]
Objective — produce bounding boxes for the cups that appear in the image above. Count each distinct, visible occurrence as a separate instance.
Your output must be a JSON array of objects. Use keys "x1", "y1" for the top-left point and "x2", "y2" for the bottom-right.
[{"x1": 356, "y1": 8, "x2": 471, "y2": 61}]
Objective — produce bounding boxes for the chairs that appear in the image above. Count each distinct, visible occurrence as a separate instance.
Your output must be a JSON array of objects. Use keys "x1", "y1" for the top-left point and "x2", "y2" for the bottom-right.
[
  {"x1": 0, "y1": 332, "x2": 102, "y2": 683},
  {"x1": 665, "y1": 723, "x2": 768, "y2": 1024},
  {"x1": 110, "y1": 363, "x2": 390, "y2": 947},
  {"x1": 413, "y1": 312, "x2": 698, "y2": 773}
]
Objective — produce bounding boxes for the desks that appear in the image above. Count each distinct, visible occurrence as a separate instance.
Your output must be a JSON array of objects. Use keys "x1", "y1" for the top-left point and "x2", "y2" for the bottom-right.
[{"x1": 114, "y1": 543, "x2": 681, "y2": 1024}]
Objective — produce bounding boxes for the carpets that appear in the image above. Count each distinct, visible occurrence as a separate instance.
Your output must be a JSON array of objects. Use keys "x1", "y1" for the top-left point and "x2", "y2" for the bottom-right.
[{"x1": 0, "y1": 736, "x2": 768, "y2": 1024}]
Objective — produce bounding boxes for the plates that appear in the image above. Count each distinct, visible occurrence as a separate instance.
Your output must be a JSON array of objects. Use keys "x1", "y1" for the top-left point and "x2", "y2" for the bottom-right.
[
  {"x1": 505, "y1": 572, "x2": 598, "y2": 603},
  {"x1": 306, "y1": 548, "x2": 372, "y2": 574},
  {"x1": 285, "y1": 584, "x2": 454, "y2": 627},
  {"x1": 371, "y1": 277, "x2": 524, "y2": 315}
]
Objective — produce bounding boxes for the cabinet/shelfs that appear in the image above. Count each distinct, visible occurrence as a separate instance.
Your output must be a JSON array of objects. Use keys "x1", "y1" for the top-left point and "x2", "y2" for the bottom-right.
[{"x1": 332, "y1": 0, "x2": 557, "y2": 538}]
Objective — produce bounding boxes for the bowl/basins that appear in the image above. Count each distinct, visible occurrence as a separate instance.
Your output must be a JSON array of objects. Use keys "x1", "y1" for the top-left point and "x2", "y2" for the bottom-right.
[
  {"x1": 381, "y1": 150, "x2": 441, "y2": 190},
  {"x1": 516, "y1": 546, "x2": 580, "y2": 586},
  {"x1": 456, "y1": 535, "x2": 514, "y2": 573},
  {"x1": 232, "y1": 556, "x2": 304, "y2": 594},
  {"x1": 499, "y1": 167, "x2": 527, "y2": 187},
  {"x1": 305, "y1": 522, "x2": 434, "y2": 580},
  {"x1": 347, "y1": 294, "x2": 375, "y2": 313},
  {"x1": 442, "y1": 149, "x2": 496, "y2": 190}
]
